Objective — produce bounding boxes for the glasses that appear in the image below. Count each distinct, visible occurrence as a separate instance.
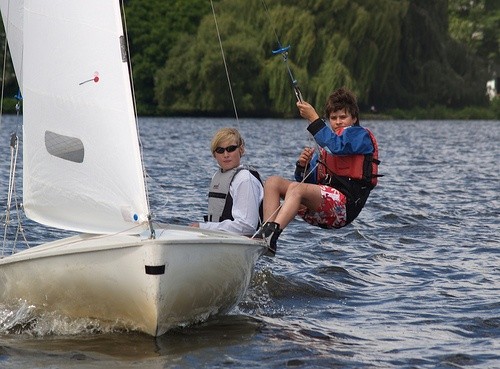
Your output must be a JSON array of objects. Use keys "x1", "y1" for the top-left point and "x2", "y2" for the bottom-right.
[{"x1": 214, "y1": 145, "x2": 238, "y2": 153}]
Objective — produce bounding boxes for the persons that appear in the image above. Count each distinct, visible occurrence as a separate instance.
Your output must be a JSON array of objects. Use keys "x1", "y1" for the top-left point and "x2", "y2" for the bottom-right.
[
  {"x1": 190, "y1": 128, "x2": 264, "y2": 238},
  {"x1": 243, "y1": 90, "x2": 378, "y2": 256}
]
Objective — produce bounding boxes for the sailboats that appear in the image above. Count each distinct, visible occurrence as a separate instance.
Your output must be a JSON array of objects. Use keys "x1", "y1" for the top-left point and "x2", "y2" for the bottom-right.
[{"x1": 0, "y1": 0, "x2": 269, "y2": 338}]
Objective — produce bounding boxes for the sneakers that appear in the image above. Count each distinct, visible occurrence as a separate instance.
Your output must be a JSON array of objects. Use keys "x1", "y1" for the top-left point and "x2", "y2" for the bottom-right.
[
  {"x1": 243, "y1": 222, "x2": 271, "y2": 240},
  {"x1": 254, "y1": 229, "x2": 283, "y2": 257}
]
